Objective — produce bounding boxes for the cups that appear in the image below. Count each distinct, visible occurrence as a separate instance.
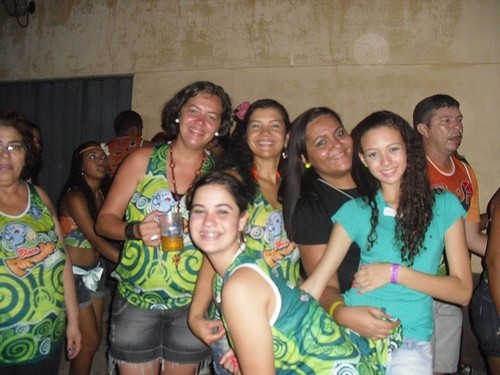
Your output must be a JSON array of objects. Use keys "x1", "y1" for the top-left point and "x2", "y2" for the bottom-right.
[{"x1": 158, "y1": 212, "x2": 184, "y2": 251}]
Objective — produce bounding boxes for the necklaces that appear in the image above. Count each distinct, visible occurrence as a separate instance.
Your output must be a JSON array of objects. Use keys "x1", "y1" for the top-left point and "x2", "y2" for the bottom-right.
[
  {"x1": 167, "y1": 141, "x2": 206, "y2": 235},
  {"x1": 250, "y1": 166, "x2": 283, "y2": 183}
]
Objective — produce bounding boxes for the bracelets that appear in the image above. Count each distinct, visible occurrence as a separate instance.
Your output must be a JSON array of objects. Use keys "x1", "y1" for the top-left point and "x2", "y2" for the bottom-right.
[
  {"x1": 390, "y1": 263, "x2": 399, "y2": 285},
  {"x1": 329, "y1": 299, "x2": 346, "y2": 319},
  {"x1": 125, "y1": 220, "x2": 141, "y2": 240}
]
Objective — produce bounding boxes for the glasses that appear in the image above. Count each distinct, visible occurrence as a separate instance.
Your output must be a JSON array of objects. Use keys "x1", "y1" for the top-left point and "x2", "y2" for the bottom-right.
[{"x1": 0, "y1": 144, "x2": 26, "y2": 155}]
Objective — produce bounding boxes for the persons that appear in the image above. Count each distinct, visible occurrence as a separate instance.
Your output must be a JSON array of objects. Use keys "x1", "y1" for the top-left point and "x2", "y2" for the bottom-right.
[
  {"x1": 186, "y1": 170, "x2": 387, "y2": 374},
  {"x1": 0, "y1": 110, "x2": 81, "y2": 375},
  {"x1": 94, "y1": 81, "x2": 234, "y2": 375},
  {"x1": 300, "y1": 110, "x2": 473, "y2": 375},
  {"x1": 413, "y1": 94, "x2": 489, "y2": 375},
  {"x1": 284, "y1": 106, "x2": 401, "y2": 340},
  {"x1": 187, "y1": 97, "x2": 307, "y2": 375},
  {"x1": 451, "y1": 150, "x2": 500, "y2": 375},
  {"x1": 100, "y1": 108, "x2": 155, "y2": 197},
  {"x1": 26, "y1": 121, "x2": 44, "y2": 176},
  {"x1": 55, "y1": 140, "x2": 126, "y2": 375}
]
